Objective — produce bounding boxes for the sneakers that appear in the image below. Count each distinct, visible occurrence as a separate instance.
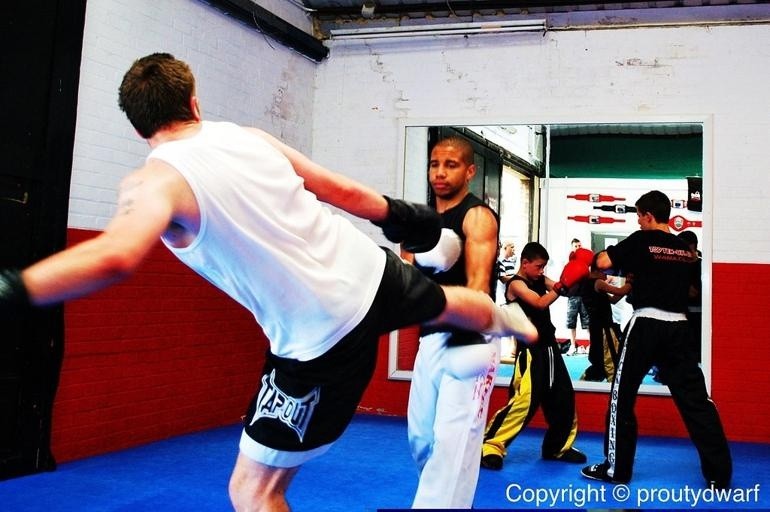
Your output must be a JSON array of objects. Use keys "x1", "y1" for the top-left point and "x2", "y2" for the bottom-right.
[
  {"x1": 481, "y1": 443, "x2": 504, "y2": 470},
  {"x1": 566, "y1": 345, "x2": 590, "y2": 356},
  {"x1": 580, "y1": 463, "x2": 631, "y2": 484},
  {"x1": 542, "y1": 447, "x2": 586, "y2": 464}
]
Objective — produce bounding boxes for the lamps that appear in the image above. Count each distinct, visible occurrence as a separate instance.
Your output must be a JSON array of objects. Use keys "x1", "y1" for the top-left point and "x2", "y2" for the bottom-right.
[{"x1": 330, "y1": 18, "x2": 547, "y2": 40}]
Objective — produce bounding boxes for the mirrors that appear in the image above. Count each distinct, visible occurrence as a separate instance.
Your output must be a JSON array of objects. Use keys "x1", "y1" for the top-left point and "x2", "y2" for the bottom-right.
[{"x1": 388, "y1": 112, "x2": 713, "y2": 398}]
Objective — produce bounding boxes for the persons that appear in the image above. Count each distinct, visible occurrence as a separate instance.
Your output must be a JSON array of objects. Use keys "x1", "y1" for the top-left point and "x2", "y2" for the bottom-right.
[
  {"x1": 480, "y1": 238, "x2": 594, "y2": 469},
  {"x1": 401, "y1": 135, "x2": 502, "y2": 511},
  {"x1": 1, "y1": 49, "x2": 547, "y2": 510},
  {"x1": 578, "y1": 187, "x2": 736, "y2": 493},
  {"x1": 565, "y1": 228, "x2": 702, "y2": 384},
  {"x1": 499, "y1": 240, "x2": 520, "y2": 358},
  {"x1": 495, "y1": 240, "x2": 516, "y2": 286}
]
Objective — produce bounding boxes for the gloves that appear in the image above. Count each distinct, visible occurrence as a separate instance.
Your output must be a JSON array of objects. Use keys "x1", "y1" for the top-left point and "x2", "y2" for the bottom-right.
[
  {"x1": 444, "y1": 329, "x2": 490, "y2": 378},
  {"x1": 370, "y1": 195, "x2": 462, "y2": 271},
  {"x1": 0, "y1": 275, "x2": 32, "y2": 319},
  {"x1": 553, "y1": 248, "x2": 594, "y2": 296}
]
